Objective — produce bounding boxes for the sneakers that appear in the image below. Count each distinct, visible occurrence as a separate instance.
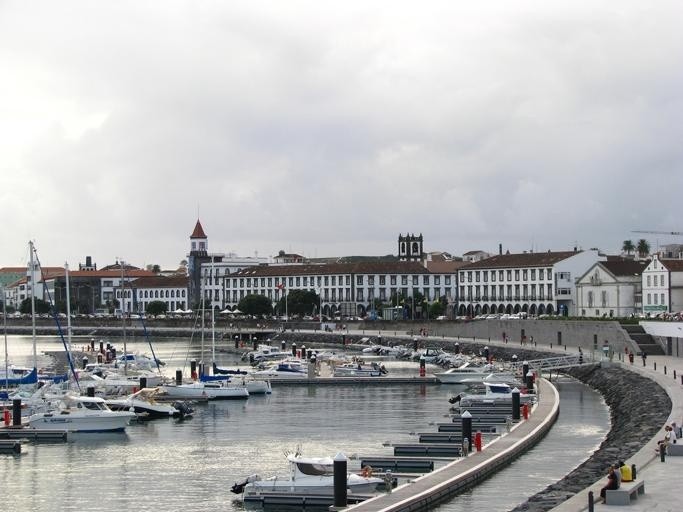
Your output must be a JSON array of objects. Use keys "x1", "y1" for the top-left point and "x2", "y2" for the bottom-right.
[
  {"x1": 655, "y1": 447, "x2": 660, "y2": 454},
  {"x1": 601, "y1": 500, "x2": 606, "y2": 504}
]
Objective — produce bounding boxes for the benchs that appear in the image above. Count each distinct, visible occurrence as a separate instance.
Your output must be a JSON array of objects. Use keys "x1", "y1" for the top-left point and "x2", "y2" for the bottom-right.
[
  {"x1": 664, "y1": 436, "x2": 681, "y2": 456},
  {"x1": 603, "y1": 477, "x2": 646, "y2": 505}
]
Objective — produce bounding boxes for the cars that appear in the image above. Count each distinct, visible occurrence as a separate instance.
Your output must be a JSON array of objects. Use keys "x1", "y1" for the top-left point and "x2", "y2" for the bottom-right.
[
  {"x1": 273, "y1": 314, "x2": 365, "y2": 322},
  {"x1": 435, "y1": 311, "x2": 529, "y2": 321}
]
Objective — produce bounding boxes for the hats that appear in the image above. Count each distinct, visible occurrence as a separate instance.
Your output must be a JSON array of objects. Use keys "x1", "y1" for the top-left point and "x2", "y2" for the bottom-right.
[{"x1": 611, "y1": 462, "x2": 619, "y2": 467}]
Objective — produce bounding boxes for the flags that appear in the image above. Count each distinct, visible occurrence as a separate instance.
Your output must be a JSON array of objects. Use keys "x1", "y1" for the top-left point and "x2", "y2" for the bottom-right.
[{"x1": 277, "y1": 283, "x2": 284, "y2": 289}]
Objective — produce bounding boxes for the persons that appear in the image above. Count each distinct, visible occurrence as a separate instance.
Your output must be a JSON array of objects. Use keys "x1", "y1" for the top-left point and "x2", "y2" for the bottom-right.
[
  {"x1": 630, "y1": 312, "x2": 634, "y2": 319},
  {"x1": 659, "y1": 427, "x2": 677, "y2": 449},
  {"x1": 654, "y1": 425, "x2": 671, "y2": 455},
  {"x1": 610, "y1": 463, "x2": 620, "y2": 487},
  {"x1": 644, "y1": 312, "x2": 683, "y2": 322},
  {"x1": 617, "y1": 459, "x2": 631, "y2": 481},
  {"x1": 599, "y1": 468, "x2": 619, "y2": 505},
  {"x1": 628, "y1": 351, "x2": 634, "y2": 362},
  {"x1": 640, "y1": 350, "x2": 647, "y2": 366},
  {"x1": 671, "y1": 422, "x2": 680, "y2": 440}
]
